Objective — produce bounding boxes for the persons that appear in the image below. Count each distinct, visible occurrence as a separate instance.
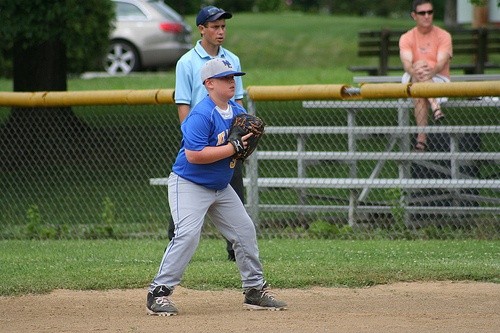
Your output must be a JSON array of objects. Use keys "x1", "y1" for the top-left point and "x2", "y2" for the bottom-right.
[
  {"x1": 146, "y1": 58, "x2": 287, "y2": 317},
  {"x1": 168, "y1": 6, "x2": 244, "y2": 261},
  {"x1": 399, "y1": 0, "x2": 453, "y2": 151}
]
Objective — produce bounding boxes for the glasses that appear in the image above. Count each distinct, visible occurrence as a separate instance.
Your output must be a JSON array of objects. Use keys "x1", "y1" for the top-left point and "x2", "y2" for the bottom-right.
[{"x1": 416, "y1": 10, "x2": 434, "y2": 15}]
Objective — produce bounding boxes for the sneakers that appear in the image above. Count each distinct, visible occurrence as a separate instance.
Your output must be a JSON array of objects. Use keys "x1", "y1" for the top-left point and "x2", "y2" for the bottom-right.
[
  {"x1": 145, "y1": 285, "x2": 178, "y2": 316},
  {"x1": 243, "y1": 281, "x2": 287, "y2": 311}
]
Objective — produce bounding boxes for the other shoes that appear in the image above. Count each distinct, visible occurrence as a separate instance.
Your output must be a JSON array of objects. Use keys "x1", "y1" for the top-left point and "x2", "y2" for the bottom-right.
[
  {"x1": 413, "y1": 136, "x2": 428, "y2": 150},
  {"x1": 432, "y1": 108, "x2": 444, "y2": 122}
]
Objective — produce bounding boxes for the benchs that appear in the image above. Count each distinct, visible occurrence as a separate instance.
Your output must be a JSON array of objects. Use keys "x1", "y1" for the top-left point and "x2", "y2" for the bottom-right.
[
  {"x1": 149, "y1": 74, "x2": 500, "y2": 225},
  {"x1": 347, "y1": 27, "x2": 500, "y2": 77}
]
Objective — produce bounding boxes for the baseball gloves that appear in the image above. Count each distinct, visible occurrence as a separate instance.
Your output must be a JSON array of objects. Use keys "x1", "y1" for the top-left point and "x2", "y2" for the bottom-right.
[{"x1": 224, "y1": 112, "x2": 265, "y2": 168}]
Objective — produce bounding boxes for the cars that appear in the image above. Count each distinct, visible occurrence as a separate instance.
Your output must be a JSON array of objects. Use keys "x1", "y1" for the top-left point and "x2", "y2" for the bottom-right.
[{"x1": 96, "y1": 0, "x2": 195, "y2": 77}]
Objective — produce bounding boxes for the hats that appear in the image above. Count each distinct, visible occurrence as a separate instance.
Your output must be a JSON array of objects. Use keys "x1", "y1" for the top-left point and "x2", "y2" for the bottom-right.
[
  {"x1": 201, "y1": 57, "x2": 246, "y2": 84},
  {"x1": 196, "y1": 6, "x2": 233, "y2": 27}
]
